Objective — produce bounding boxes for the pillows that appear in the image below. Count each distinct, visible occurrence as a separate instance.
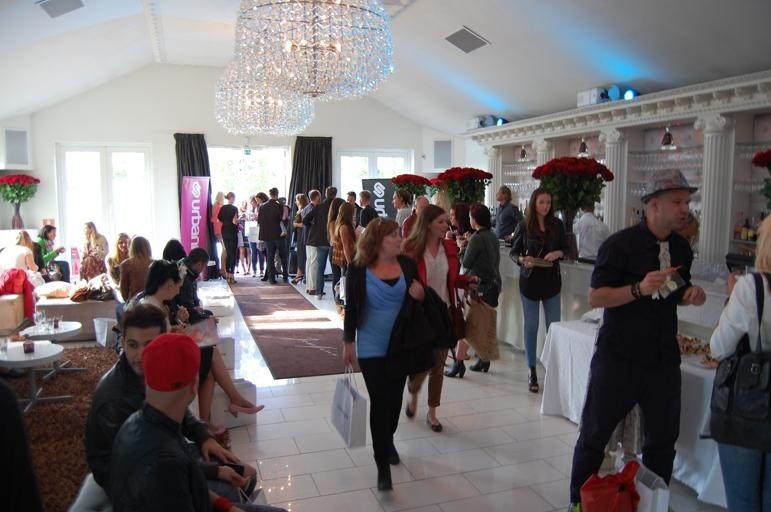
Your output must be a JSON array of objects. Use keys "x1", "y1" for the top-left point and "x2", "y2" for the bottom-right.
[{"x1": 34, "y1": 281, "x2": 74, "y2": 297}]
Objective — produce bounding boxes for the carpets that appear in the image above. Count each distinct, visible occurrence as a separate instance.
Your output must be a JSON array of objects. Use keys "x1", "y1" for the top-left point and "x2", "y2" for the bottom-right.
[
  {"x1": 0, "y1": 346, "x2": 120, "y2": 512},
  {"x1": 228, "y1": 276, "x2": 361, "y2": 380}
]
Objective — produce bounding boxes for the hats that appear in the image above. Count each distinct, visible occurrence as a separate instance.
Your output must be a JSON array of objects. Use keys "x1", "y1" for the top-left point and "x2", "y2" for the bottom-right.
[
  {"x1": 143, "y1": 334, "x2": 201, "y2": 392},
  {"x1": 640, "y1": 169, "x2": 697, "y2": 203}
]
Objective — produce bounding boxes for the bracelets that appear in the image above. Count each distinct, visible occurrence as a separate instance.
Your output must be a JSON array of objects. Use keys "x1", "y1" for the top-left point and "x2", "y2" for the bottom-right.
[
  {"x1": 213, "y1": 496, "x2": 235, "y2": 510},
  {"x1": 632, "y1": 283, "x2": 641, "y2": 299},
  {"x1": 638, "y1": 281, "x2": 644, "y2": 298}
]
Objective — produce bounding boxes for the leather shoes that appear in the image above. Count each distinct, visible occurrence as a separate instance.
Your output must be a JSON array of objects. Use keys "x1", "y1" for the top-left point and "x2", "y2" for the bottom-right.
[
  {"x1": 426, "y1": 415, "x2": 443, "y2": 431},
  {"x1": 405, "y1": 402, "x2": 417, "y2": 417}
]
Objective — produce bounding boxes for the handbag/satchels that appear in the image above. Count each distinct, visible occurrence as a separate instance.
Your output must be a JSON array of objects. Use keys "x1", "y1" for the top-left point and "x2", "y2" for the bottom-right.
[
  {"x1": 330, "y1": 364, "x2": 367, "y2": 448},
  {"x1": 580, "y1": 453, "x2": 669, "y2": 512},
  {"x1": 710, "y1": 353, "x2": 771, "y2": 451},
  {"x1": 239, "y1": 484, "x2": 267, "y2": 506},
  {"x1": 460, "y1": 288, "x2": 499, "y2": 360}
]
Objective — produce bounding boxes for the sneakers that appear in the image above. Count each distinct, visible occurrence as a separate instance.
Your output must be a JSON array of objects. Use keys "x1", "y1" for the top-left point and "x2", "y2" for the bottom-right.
[
  {"x1": 387, "y1": 441, "x2": 400, "y2": 464},
  {"x1": 570, "y1": 502, "x2": 581, "y2": 511},
  {"x1": 307, "y1": 289, "x2": 326, "y2": 299},
  {"x1": 377, "y1": 469, "x2": 392, "y2": 491}
]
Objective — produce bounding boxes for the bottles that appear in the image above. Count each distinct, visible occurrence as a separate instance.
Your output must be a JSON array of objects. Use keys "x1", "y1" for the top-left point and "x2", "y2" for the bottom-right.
[
  {"x1": 503, "y1": 161, "x2": 538, "y2": 177},
  {"x1": 505, "y1": 182, "x2": 537, "y2": 196},
  {"x1": 630, "y1": 207, "x2": 647, "y2": 231},
  {"x1": 734, "y1": 212, "x2": 771, "y2": 243}
]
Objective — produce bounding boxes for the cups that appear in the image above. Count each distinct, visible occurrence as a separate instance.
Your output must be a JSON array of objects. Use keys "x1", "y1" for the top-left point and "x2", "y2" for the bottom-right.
[
  {"x1": 33, "y1": 309, "x2": 47, "y2": 329},
  {"x1": 0, "y1": 338, "x2": 8, "y2": 354}
]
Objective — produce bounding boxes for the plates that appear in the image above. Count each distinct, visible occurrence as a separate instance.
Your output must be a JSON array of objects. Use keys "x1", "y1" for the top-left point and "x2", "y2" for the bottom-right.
[{"x1": 524, "y1": 259, "x2": 554, "y2": 267}]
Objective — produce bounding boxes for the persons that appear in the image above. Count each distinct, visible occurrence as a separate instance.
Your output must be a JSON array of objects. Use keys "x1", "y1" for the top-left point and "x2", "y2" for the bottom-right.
[
  {"x1": 343, "y1": 220, "x2": 426, "y2": 494},
  {"x1": 206, "y1": 186, "x2": 608, "y2": 379},
  {"x1": 509, "y1": 190, "x2": 571, "y2": 392},
  {"x1": 106, "y1": 333, "x2": 292, "y2": 511},
  {"x1": 709, "y1": 211, "x2": 771, "y2": 512},
  {"x1": 85, "y1": 305, "x2": 257, "y2": 503},
  {"x1": 401, "y1": 203, "x2": 482, "y2": 431},
  {"x1": 2, "y1": 220, "x2": 219, "y2": 336},
  {"x1": 674, "y1": 211, "x2": 700, "y2": 245},
  {"x1": 127, "y1": 257, "x2": 265, "y2": 437},
  {"x1": 569, "y1": 168, "x2": 706, "y2": 512}
]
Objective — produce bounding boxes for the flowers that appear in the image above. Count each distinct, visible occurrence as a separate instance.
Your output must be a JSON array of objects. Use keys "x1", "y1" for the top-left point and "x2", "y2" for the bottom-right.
[
  {"x1": 392, "y1": 167, "x2": 493, "y2": 205},
  {"x1": 531, "y1": 157, "x2": 614, "y2": 262},
  {"x1": 0, "y1": 175, "x2": 40, "y2": 228}
]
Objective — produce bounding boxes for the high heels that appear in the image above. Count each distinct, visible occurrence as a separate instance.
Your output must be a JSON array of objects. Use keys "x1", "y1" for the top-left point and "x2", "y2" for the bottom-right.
[
  {"x1": 230, "y1": 404, "x2": 265, "y2": 418},
  {"x1": 444, "y1": 360, "x2": 466, "y2": 378},
  {"x1": 217, "y1": 270, "x2": 237, "y2": 284},
  {"x1": 291, "y1": 276, "x2": 304, "y2": 285},
  {"x1": 469, "y1": 358, "x2": 490, "y2": 372},
  {"x1": 212, "y1": 426, "x2": 225, "y2": 434}
]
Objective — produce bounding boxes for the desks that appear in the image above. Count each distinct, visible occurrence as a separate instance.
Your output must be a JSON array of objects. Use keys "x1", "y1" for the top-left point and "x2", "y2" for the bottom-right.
[
  {"x1": 540, "y1": 320, "x2": 728, "y2": 509},
  {"x1": 0, "y1": 321, "x2": 87, "y2": 416}
]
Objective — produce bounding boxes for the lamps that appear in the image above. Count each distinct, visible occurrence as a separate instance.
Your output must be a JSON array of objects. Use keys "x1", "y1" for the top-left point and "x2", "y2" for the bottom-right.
[{"x1": 213, "y1": 0, "x2": 396, "y2": 136}]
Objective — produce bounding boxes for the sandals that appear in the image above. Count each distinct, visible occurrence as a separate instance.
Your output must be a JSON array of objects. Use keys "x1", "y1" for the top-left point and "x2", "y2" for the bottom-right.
[{"x1": 527, "y1": 370, "x2": 539, "y2": 393}]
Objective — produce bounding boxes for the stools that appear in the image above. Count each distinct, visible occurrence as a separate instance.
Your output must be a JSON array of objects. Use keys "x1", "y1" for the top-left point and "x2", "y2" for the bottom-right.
[
  {"x1": 0, "y1": 293, "x2": 117, "y2": 342},
  {"x1": 68, "y1": 473, "x2": 114, "y2": 512}
]
